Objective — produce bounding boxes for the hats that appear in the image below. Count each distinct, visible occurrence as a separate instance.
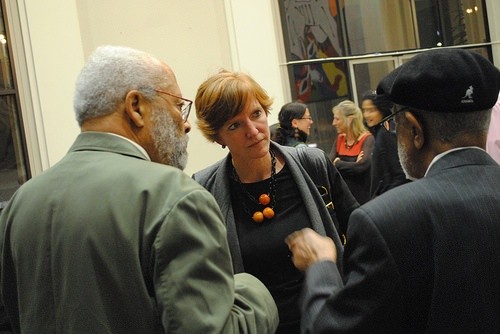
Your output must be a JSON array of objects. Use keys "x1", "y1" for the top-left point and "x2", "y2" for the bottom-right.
[{"x1": 377, "y1": 48, "x2": 500, "y2": 113}]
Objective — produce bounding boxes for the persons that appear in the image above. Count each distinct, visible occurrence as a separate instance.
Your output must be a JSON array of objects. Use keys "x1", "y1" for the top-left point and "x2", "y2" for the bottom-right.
[
  {"x1": 272, "y1": 102, "x2": 313, "y2": 147},
  {"x1": 328, "y1": 100, "x2": 375, "y2": 205},
  {"x1": 362, "y1": 91, "x2": 392, "y2": 136},
  {"x1": 189, "y1": 72, "x2": 361, "y2": 334},
  {"x1": 370, "y1": 93, "x2": 412, "y2": 200},
  {"x1": 285, "y1": 49, "x2": 500, "y2": 334},
  {"x1": 0, "y1": 44, "x2": 280, "y2": 333}
]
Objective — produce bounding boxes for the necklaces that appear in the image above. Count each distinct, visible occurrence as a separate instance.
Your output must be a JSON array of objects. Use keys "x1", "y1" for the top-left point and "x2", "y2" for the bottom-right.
[{"x1": 228, "y1": 147, "x2": 277, "y2": 222}]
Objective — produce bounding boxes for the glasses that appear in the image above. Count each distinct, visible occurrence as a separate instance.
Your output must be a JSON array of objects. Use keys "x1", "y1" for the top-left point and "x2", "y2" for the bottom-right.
[
  {"x1": 154, "y1": 89, "x2": 193, "y2": 122},
  {"x1": 299, "y1": 116, "x2": 312, "y2": 122},
  {"x1": 379, "y1": 106, "x2": 410, "y2": 135}
]
